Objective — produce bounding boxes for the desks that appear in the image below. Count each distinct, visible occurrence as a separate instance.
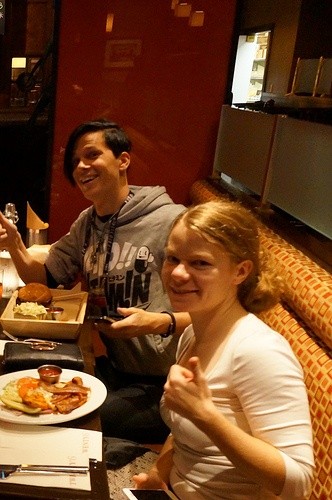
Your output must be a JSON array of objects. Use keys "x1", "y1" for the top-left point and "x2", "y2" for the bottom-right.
[{"x1": 0, "y1": 244, "x2": 111, "y2": 500}]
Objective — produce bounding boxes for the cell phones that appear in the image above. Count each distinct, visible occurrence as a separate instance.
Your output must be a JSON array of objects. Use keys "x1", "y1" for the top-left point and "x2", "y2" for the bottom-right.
[
  {"x1": 87, "y1": 314, "x2": 115, "y2": 324},
  {"x1": 122, "y1": 487, "x2": 179, "y2": 500}
]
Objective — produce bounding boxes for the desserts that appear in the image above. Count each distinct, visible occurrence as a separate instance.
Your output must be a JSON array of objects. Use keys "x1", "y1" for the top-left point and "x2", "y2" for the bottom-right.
[
  {"x1": 13, "y1": 301, "x2": 47, "y2": 320},
  {"x1": 17, "y1": 282, "x2": 52, "y2": 307}
]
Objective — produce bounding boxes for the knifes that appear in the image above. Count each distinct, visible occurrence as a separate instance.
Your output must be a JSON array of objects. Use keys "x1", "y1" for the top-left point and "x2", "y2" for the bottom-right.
[{"x1": 0, "y1": 463, "x2": 89, "y2": 476}]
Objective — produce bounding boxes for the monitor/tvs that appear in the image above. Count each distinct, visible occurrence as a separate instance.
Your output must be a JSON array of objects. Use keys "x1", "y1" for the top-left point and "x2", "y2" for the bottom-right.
[{"x1": 227, "y1": 23, "x2": 272, "y2": 107}]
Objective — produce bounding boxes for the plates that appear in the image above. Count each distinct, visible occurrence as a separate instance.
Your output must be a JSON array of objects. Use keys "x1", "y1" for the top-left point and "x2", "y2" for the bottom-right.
[{"x1": 0, "y1": 369, "x2": 107, "y2": 425}]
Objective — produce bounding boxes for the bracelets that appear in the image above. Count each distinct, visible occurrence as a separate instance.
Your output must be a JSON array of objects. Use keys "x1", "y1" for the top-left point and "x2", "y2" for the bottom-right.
[{"x1": 161, "y1": 311, "x2": 177, "y2": 338}]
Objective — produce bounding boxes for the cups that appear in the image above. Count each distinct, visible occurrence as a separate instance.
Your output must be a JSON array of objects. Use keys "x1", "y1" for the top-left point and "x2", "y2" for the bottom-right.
[
  {"x1": 47, "y1": 306, "x2": 64, "y2": 320},
  {"x1": 37, "y1": 364, "x2": 62, "y2": 384}
]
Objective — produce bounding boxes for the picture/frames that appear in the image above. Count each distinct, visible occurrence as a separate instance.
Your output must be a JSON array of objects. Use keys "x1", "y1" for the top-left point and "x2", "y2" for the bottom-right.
[{"x1": 104, "y1": 39, "x2": 142, "y2": 69}]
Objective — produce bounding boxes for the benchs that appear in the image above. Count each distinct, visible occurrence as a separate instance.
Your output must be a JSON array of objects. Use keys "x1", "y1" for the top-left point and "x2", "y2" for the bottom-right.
[{"x1": 137, "y1": 181, "x2": 332, "y2": 500}]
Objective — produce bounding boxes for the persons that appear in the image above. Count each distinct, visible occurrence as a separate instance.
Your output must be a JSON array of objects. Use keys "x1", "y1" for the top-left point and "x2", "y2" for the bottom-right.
[
  {"x1": 0, "y1": 118, "x2": 192, "y2": 444},
  {"x1": 132, "y1": 199, "x2": 314, "y2": 500}
]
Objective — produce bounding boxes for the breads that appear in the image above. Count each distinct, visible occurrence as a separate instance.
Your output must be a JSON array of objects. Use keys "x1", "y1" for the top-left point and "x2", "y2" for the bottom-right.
[{"x1": 0, "y1": 377, "x2": 67, "y2": 415}]
